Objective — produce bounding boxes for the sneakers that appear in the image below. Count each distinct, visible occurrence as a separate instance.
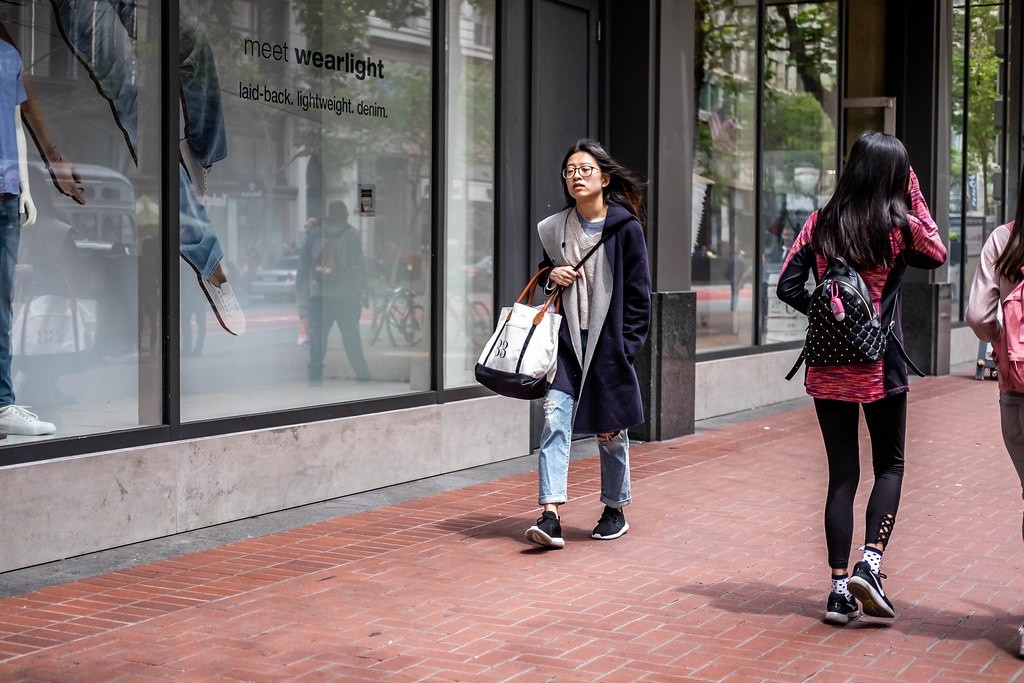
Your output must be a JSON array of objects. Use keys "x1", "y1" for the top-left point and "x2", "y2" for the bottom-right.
[
  {"x1": 848, "y1": 561, "x2": 896, "y2": 618},
  {"x1": 525, "y1": 510, "x2": 565, "y2": 548},
  {"x1": 200, "y1": 275, "x2": 246, "y2": 336},
  {"x1": 825, "y1": 591, "x2": 860, "y2": 624},
  {"x1": 592, "y1": 505, "x2": 630, "y2": 539},
  {"x1": 0, "y1": 405, "x2": 57, "y2": 434}
]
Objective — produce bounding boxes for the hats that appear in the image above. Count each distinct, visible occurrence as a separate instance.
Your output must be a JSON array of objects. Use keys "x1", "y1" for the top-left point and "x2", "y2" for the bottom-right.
[{"x1": 322, "y1": 199, "x2": 351, "y2": 223}]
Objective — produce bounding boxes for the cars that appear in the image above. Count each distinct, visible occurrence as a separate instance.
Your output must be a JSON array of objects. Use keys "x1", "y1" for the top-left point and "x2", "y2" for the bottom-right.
[
  {"x1": 249, "y1": 256, "x2": 301, "y2": 303},
  {"x1": 451, "y1": 255, "x2": 492, "y2": 292}
]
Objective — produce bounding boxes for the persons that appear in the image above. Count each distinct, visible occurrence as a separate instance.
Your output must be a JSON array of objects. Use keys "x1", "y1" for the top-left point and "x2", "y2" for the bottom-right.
[
  {"x1": 0, "y1": 0, "x2": 246, "y2": 336},
  {"x1": 964, "y1": 165, "x2": 1024, "y2": 541},
  {"x1": 775, "y1": 130, "x2": 947, "y2": 622},
  {"x1": 297, "y1": 201, "x2": 371, "y2": 386},
  {"x1": 525, "y1": 140, "x2": 651, "y2": 547},
  {"x1": 723, "y1": 249, "x2": 744, "y2": 311},
  {"x1": 0, "y1": 40, "x2": 58, "y2": 437},
  {"x1": 761, "y1": 231, "x2": 772, "y2": 334},
  {"x1": 101, "y1": 238, "x2": 205, "y2": 358}
]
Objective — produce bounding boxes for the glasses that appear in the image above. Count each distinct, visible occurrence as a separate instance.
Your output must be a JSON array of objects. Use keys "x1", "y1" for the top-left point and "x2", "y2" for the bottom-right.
[{"x1": 563, "y1": 165, "x2": 608, "y2": 179}]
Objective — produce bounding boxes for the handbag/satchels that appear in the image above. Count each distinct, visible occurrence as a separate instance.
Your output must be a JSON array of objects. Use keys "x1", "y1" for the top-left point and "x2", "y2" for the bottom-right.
[
  {"x1": 297, "y1": 315, "x2": 314, "y2": 350},
  {"x1": 8, "y1": 265, "x2": 100, "y2": 360},
  {"x1": 475, "y1": 268, "x2": 564, "y2": 400}
]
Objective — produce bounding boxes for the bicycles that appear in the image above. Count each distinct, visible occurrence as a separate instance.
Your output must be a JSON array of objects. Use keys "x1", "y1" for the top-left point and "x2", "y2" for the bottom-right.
[
  {"x1": 367, "y1": 287, "x2": 424, "y2": 348},
  {"x1": 449, "y1": 293, "x2": 493, "y2": 349}
]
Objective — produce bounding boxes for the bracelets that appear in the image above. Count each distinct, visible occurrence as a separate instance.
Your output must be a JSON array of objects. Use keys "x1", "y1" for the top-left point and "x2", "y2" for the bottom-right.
[
  {"x1": 49, "y1": 154, "x2": 63, "y2": 170},
  {"x1": 47, "y1": 147, "x2": 57, "y2": 162}
]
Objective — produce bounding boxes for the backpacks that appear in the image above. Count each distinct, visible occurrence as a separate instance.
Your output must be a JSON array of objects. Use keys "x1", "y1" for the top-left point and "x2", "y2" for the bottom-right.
[
  {"x1": 801, "y1": 255, "x2": 889, "y2": 366},
  {"x1": 999, "y1": 267, "x2": 1024, "y2": 393}
]
